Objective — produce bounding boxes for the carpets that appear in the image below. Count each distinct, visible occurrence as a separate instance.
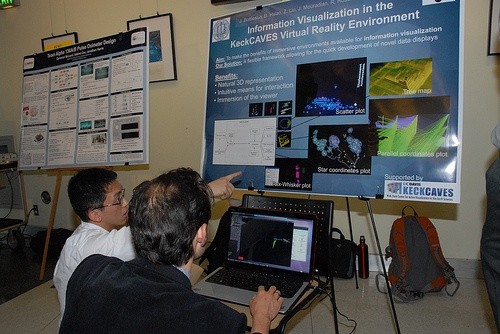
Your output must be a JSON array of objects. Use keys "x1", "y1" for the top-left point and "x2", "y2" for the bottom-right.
[{"x1": 0, "y1": 225, "x2": 74, "y2": 304}]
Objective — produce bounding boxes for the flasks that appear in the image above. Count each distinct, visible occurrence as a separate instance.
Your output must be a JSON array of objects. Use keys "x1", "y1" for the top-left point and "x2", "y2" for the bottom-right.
[{"x1": 358, "y1": 235, "x2": 369, "y2": 279}]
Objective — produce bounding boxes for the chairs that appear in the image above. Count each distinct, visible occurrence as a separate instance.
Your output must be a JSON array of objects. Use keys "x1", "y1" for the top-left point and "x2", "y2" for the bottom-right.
[{"x1": 198, "y1": 194, "x2": 339, "y2": 334}]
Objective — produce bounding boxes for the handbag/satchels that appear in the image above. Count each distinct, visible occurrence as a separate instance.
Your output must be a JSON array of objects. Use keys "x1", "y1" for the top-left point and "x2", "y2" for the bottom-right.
[{"x1": 325, "y1": 227, "x2": 359, "y2": 279}]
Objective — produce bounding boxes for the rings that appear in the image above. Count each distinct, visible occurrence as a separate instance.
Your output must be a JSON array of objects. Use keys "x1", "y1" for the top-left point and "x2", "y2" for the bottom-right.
[{"x1": 276, "y1": 291, "x2": 280, "y2": 295}]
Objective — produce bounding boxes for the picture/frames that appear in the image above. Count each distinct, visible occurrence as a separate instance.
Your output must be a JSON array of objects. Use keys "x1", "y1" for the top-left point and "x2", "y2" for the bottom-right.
[{"x1": 127, "y1": 12, "x2": 178, "y2": 83}]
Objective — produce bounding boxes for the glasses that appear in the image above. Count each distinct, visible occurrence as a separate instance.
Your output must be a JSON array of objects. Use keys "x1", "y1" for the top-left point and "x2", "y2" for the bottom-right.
[{"x1": 90, "y1": 189, "x2": 126, "y2": 212}]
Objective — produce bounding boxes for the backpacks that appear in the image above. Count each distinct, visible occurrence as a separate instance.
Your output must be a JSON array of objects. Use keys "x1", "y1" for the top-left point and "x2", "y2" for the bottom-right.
[{"x1": 386, "y1": 206, "x2": 460, "y2": 304}]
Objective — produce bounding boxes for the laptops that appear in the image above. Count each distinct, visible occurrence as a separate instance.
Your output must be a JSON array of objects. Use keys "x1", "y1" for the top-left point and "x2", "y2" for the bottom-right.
[{"x1": 189, "y1": 205, "x2": 315, "y2": 314}]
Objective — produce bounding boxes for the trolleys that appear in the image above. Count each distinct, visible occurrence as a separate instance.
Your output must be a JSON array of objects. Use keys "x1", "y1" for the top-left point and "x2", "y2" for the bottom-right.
[{"x1": 0, "y1": 160, "x2": 29, "y2": 252}]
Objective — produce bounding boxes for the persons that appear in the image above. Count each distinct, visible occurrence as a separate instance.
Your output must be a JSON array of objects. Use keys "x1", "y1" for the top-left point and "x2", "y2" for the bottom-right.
[
  {"x1": 53, "y1": 168, "x2": 241, "y2": 334},
  {"x1": 59, "y1": 168, "x2": 282, "y2": 334}
]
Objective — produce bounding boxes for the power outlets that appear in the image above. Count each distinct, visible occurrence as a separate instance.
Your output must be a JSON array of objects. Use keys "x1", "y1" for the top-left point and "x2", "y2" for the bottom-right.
[{"x1": 33, "y1": 205, "x2": 39, "y2": 216}]
